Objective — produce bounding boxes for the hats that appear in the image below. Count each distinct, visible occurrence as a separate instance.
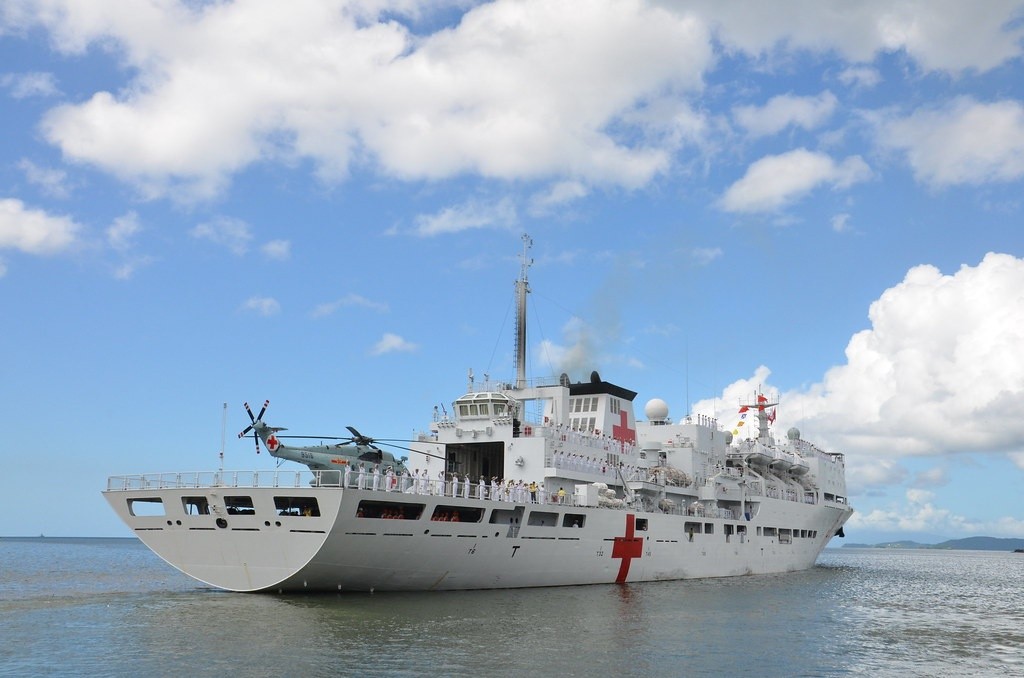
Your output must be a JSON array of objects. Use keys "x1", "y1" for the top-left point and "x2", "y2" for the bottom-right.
[
  {"x1": 375, "y1": 464, "x2": 378, "y2": 468},
  {"x1": 424, "y1": 469, "x2": 427, "y2": 472},
  {"x1": 387, "y1": 466, "x2": 391, "y2": 469},
  {"x1": 361, "y1": 463, "x2": 364, "y2": 467},
  {"x1": 441, "y1": 470, "x2": 444, "y2": 473},
  {"x1": 480, "y1": 475, "x2": 484, "y2": 478},
  {"x1": 346, "y1": 460, "x2": 350, "y2": 463},
  {"x1": 492, "y1": 477, "x2": 495, "y2": 480},
  {"x1": 466, "y1": 474, "x2": 469, "y2": 477},
  {"x1": 453, "y1": 472, "x2": 457, "y2": 475},
  {"x1": 403, "y1": 467, "x2": 407, "y2": 470}
]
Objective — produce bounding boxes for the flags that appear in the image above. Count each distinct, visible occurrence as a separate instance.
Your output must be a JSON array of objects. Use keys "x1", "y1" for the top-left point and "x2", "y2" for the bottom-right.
[
  {"x1": 733, "y1": 429, "x2": 738, "y2": 435},
  {"x1": 741, "y1": 414, "x2": 747, "y2": 419},
  {"x1": 737, "y1": 421, "x2": 745, "y2": 427}
]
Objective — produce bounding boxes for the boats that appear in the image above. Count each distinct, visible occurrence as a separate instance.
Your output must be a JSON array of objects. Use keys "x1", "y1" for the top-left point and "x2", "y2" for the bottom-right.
[
  {"x1": 102, "y1": 233, "x2": 855, "y2": 593},
  {"x1": 689, "y1": 502, "x2": 705, "y2": 513},
  {"x1": 592, "y1": 482, "x2": 624, "y2": 508},
  {"x1": 658, "y1": 499, "x2": 675, "y2": 511}
]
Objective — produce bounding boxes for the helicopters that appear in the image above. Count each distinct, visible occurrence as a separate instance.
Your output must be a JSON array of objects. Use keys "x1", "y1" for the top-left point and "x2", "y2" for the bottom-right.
[{"x1": 237, "y1": 399, "x2": 462, "y2": 492}]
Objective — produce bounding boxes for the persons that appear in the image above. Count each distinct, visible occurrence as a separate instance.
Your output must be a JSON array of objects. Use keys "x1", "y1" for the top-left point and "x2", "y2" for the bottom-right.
[
  {"x1": 794, "y1": 438, "x2": 832, "y2": 460},
  {"x1": 491, "y1": 476, "x2": 546, "y2": 504},
  {"x1": 697, "y1": 413, "x2": 718, "y2": 430},
  {"x1": 430, "y1": 511, "x2": 459, "y2": 522},
  {"x1": 689, "y1": 528, "x2": 694, "y2": 534},
  {"x1": 573, "y1": 520, "x2": 578, "y2": 528},
  {"x1": 303, "y1": 506, "x2": 312, "y2": 517},
  {"x1": 557, "y1": 487, "x2": 566, "y2": 506},
  {"x1": 760, "y1": 442, "x2": 794, "y2": 463},
  {"x1": 401, "y1": 468, "x2": 445, "y2": 496},
  {"x1": 767, "y1": 487, "x2": 813, "y2": 504},
  {"x1": 552, "y1": 449, "x2": 637, "y2": 481},
  {"x1": 556, "y1": 424, "x2": 636, "y2": 455},
  {"x1": 357, "y1": 508, "x2": 365, "y2": 518},
  {"x1": 450, "y1": 467, "x2": 485, "y2": 501},
  {"x1": 343, "y1": 460, "x2": 394, "y2": 493},
  {"x1": 381, "y1": 508, "x2": 421, "y2": 520}
]
